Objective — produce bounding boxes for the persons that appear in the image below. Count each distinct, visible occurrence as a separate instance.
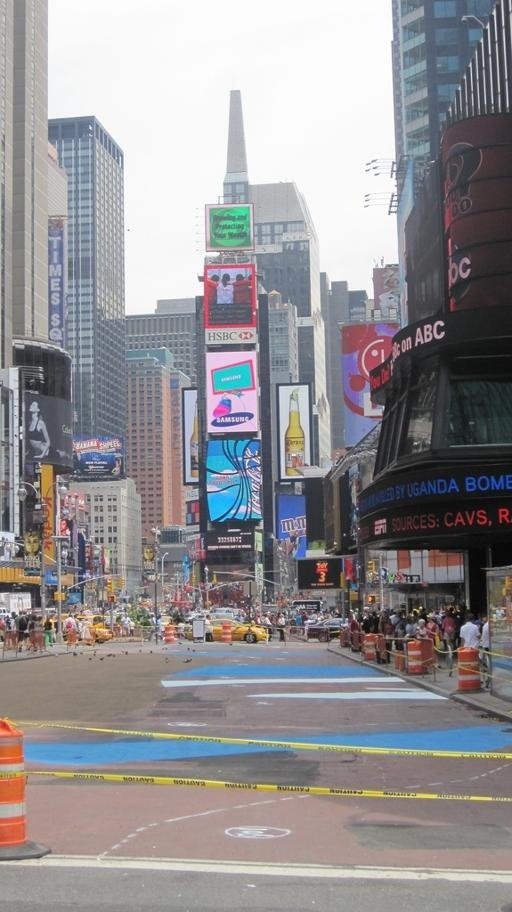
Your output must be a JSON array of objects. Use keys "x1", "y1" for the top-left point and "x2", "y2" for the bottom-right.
[
  {"x1": 234, "y1": 273, "x2": 249, "y2": 304},
  {"x1": 150, "y1": 614, "x2": 214, "y2": 642},
  {"x1": 207, "y1": 273, "x2": 248, "y2": 304},
  {"x1": 247, "y1": 275, "x2": 252, "y2": 304},
  {"x1": 1, "y1": 604, "x2": 94, "y2": 646},
  {"x1": 121, "y1": 614, "x2": 135, "y2": 635},
  {"x1": 238, "y1": 606, "x2": 338, "y2": 642},
  {"x1": 208, "y1": 274, "x2": 220, "y2": 304},
  {"x1": 27, "y1": 400, "x2": 67, "y2": 460},
  {"x1": 343, "y1": 602, "x2": 491, "y2": 686}
]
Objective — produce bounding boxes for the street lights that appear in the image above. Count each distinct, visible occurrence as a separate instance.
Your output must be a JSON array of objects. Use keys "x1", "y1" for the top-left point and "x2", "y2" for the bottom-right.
[
  {"x1": 162, "y1": 552, "x2": 169, "y2": 585},
  {"x1": 14, "y1": 480, "x2": 67, "y2": 613},
  {"x1": 346, "y1": 576, "x2": 353, "y2": 618},
  {"x1": 56, "y1": 474, "x2": 70, "y2": 644},
  {"x1": 151, "y1": 525, "x2": 161, "y2": 621},
  {"x1": 203, "y1": 564, "x2": 209, "y2": 609}
]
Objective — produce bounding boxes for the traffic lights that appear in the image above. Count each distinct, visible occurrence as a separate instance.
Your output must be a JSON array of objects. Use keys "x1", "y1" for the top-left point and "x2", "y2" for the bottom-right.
[
  {"x1": 54, "y1": 592, "x2": 59, "y2": 600},
  {"x1": 118, "y1": 577, "x2": 125, "y2": 587},
  {"x1": 107, "y1": 581, "x2": 112, "y2": 591},
  {"x1": 367, "y1": 561, "x2": 375, "y2": 571}
]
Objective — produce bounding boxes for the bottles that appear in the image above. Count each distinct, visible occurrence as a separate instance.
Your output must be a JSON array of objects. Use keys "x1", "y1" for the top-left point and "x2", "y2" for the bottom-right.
[{"x1": 283, "y1": 387, "x2": 306, "y2": 477}]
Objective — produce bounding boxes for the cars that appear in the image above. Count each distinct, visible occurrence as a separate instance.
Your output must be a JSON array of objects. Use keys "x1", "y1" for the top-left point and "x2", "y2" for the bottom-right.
[
  {"x1": 307, "y1": 618, "x2": 344, "y2": 641},
  {"x1": 184, "y1": 608, "x2": 267, "y2": 644},
  {"x1": 33, "y1": 607, "x2": 113, "y2": 643}
]
